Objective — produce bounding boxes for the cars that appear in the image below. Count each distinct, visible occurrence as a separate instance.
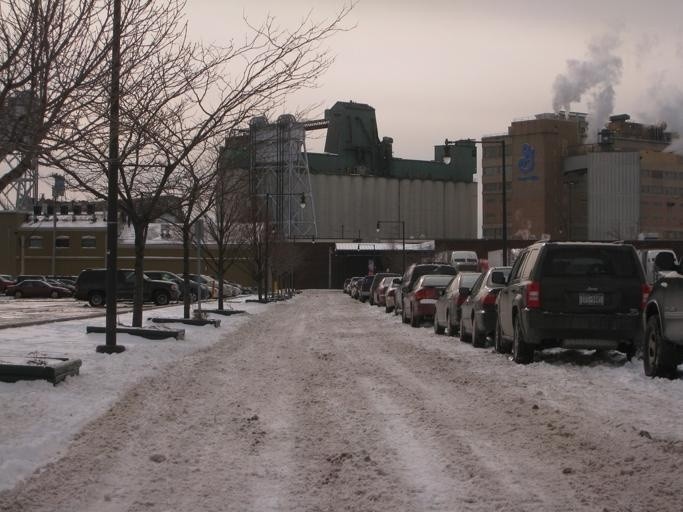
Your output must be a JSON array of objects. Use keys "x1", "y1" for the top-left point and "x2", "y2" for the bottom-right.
[
  {"x1": 641, "y1": 254, "x2": 682, "y2": 382},
  {"x1": 0, "y1": 267, "x2": 251, "y2": 307}
]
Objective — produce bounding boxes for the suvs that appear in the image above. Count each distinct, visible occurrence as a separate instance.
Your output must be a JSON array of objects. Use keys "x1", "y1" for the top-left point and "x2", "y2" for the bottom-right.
[{"x1": 491, "y1": 235, "x2": 654, "y2": 369}]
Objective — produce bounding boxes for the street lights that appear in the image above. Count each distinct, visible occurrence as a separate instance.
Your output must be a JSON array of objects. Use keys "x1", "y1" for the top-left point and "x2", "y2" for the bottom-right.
[
  {"x1": 440, "y1": 134, "x2": 509, "y2": 270},
  {"x1": 288, "y1": 233, "x2": 318, "y2": 295},
  {"x1": 376, "y1": 220, "x2": 405, "y2": 275},
  {"x1": 263, "y1": 188, "x2": 308, "y2": 303}
]
